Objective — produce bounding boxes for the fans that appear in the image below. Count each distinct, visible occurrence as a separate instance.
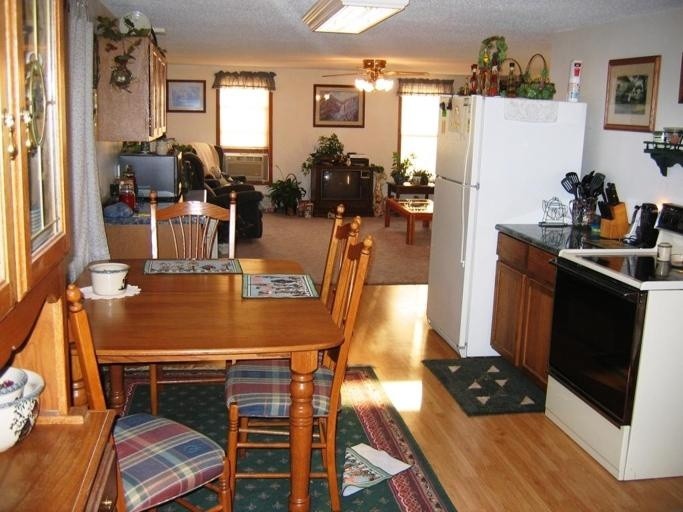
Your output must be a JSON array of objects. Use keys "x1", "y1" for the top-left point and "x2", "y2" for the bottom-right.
[{"x1": 319, "y1": 57, "x2": 431, "y2": 83}]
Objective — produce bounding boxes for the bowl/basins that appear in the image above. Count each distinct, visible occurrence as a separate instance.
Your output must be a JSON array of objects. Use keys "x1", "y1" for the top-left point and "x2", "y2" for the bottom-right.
[
  {"x1": 0, "y1": 366, "x2": 47, "y2": 454},
  {"x1": 87, "y1": 261, "x2": 129, "y2": 295}
]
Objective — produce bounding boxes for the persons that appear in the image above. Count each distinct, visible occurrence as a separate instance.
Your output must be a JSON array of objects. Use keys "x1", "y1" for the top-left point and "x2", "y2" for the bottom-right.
[{"x1": 620, "y1": 75, "x2": 647, "y2": 104}]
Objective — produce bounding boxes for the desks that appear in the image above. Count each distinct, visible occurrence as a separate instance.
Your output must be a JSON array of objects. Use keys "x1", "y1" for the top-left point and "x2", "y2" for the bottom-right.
[
  {"x1": 103, "y1": 189, "x2": 207, "y2": 260},
  {"x1": 384, "y1": 197, "x2": 433, "y2": 245},
  {"x1": 73, "y1": 256, "x2": 347, "y2": 512}
]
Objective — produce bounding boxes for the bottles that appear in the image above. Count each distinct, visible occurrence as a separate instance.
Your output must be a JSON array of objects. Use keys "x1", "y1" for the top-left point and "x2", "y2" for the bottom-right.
[
  {"x1": 463, "y1": 48, "x2": 516, "y2": 97},
  {"x1": 567, "y1": 60, "x2": 583, "y2": 101},
  {"x1": 120, "y1": 164, "x2": 140, "y2": 212}
]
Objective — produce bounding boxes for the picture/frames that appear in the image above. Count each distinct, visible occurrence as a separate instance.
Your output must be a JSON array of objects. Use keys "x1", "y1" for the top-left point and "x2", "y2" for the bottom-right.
[
  {"x1": 164, "y1": 78, "x2": 206, "y2": 114},
  {"x1": 312, "y1": 83, "x2": 365, "y2": 129},
  {"x1": 600, "y1": 54, "x2": 661, "y2": 134}
]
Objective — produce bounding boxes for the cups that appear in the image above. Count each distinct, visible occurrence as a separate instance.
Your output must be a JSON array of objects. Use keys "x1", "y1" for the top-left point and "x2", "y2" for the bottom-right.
[
  {"x1": 655, "y1": 262, "x2": 670, "y2": 279},
  {"x1": 654, "y1": 241, "x2": 670, "y2": 262},
  {"x1": 567, "y1": 199, "x2": 596, "y2": 230}
]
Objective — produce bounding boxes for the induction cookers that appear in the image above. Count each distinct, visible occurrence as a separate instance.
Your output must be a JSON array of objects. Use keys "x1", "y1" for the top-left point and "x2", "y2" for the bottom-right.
[{"x1": 558, "y1": 202, "x2": 683, "y2": 291}]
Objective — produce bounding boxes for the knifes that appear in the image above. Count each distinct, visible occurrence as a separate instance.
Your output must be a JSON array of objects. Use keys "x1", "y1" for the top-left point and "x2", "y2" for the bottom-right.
[{"x1": 598, "y1": 182, "x2": 619, "y2": 219}]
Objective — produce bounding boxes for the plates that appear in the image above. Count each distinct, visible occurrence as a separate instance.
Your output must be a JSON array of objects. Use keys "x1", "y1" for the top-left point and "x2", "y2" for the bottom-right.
[{"x1": 118, "y1": 12, "x2": 151, "y2": 37}]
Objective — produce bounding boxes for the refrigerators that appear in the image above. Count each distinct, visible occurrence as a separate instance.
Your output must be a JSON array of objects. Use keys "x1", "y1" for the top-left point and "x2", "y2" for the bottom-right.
[{"x1": 423, "y1": 95, "x2": 590, "y2": 356}]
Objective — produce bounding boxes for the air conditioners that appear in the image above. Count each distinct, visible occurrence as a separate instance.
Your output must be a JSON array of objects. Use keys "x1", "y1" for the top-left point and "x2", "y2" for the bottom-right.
[{"x1": 222, "y1": 152, "x2": 269, "y2": 183}]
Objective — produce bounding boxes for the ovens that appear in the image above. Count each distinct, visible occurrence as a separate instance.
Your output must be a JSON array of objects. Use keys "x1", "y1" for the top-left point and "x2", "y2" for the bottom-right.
[{"x1": 544, "y1": 257, "x2": 645, "y2": 427}]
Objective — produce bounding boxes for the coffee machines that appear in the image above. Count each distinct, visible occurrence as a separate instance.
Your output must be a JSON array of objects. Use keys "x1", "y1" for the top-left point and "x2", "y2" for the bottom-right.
[{"x1": 622, "y1": 202, "x2": 658, "y2": 247}]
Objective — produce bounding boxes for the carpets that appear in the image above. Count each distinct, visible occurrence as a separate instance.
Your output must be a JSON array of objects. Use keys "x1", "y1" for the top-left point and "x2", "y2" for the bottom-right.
[
  {"x1": 420, "y1": 355, "x2": 547, "y2": 418},
  {"x1": 100, "y1": 363, "x2": 458, "y2": 510},
  {"x1": 218, "y1": 211, "x2": 432, "y2": 286}
]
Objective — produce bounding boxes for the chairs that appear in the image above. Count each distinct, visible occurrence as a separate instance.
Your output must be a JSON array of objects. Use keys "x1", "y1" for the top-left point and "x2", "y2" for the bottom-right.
[
  {"x1": 224, "y1": 202, "x2": 363, "y2": 370},
  {"x1": 222, "y1": 220, "x2": 372, "y2": 512},
  {"x1": 147, "y1": 187, "x2": 239, "y2": 415},
  {"x1": 184, "y1": 142, "x2": 248, "y2": 185},
  {"x1": 179, "y1": 142, "x2": 264, "y2": 245},
  {"x1": 66, "y1": 282, "x2": 233, "y2": 512}
]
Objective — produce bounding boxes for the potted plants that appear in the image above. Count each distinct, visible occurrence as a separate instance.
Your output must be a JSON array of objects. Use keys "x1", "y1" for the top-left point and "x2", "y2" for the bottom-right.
[{"x1": 264, "y1": 132, "x2": 433, "y2": 221}]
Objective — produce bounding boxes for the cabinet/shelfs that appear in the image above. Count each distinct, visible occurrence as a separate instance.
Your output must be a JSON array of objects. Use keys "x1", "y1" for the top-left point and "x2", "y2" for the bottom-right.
[
  {"x1": 94, "y1": 34, "x2": 167, "y2": 142},
  {"x1": 0, "y1": 0, "x2": 127, "y2": 512},
  {"x1": 488, "y1": 219, "x2": 639, "y2": 395}
]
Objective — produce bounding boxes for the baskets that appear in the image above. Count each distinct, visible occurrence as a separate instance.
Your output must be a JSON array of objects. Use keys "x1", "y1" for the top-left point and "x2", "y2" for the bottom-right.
[
  {"x1": 498, "y1": 58, "x2": 523, "y2": 97},
  {"x1": 524, "y1": 53, "x2": 555, "y2": 98}
]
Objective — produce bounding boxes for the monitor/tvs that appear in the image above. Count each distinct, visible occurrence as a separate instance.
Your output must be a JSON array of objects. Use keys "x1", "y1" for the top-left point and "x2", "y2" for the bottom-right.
[{"x1": 321, "y1": 168, "x2": 361, "y2": 200}]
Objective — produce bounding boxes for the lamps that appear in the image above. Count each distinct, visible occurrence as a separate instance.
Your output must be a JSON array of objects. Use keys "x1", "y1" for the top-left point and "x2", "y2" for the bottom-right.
[{"x1": 301, "y1": 1, "x2": 409, "y2": 35}]
